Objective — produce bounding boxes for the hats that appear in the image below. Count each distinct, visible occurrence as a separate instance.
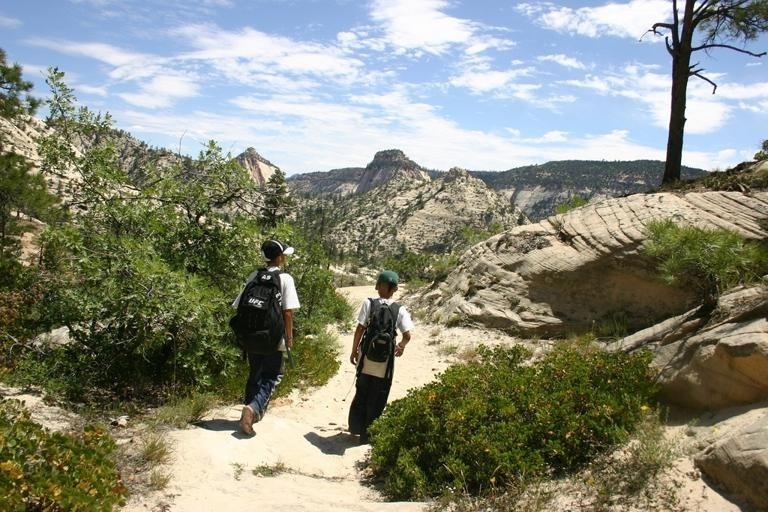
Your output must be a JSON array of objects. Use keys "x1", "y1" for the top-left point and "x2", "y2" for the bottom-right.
[
  {"x1": 260, "y1": 239, "x2": 295, "y2": 263},
  {"x1": 375, "y1": 270, "x2": 399, "y2": 290}
]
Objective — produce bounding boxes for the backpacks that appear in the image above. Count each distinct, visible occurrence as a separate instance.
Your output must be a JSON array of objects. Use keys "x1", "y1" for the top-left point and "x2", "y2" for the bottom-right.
[
  {"x1": 229, "y1": 268, "x2": 285, "y2": 356},
  {"x1": 360, "y1": 297, "x2": 401, "y2": 363}
]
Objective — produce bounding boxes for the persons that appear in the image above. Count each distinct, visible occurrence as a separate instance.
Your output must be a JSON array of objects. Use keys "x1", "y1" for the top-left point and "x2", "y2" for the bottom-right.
[
  {"x1": 349, "y1": 271, "x2": 412, "y2": 442},
  {"x1": 232, "y1": 240, "x2": 300, "y2": 436}
]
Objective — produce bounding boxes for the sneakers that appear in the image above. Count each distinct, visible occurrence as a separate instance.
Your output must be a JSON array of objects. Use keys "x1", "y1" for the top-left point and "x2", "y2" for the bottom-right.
[
  {"x1": 348, "y1": 426, "x2": 368, "y2": 444},
  {"x1": 238, "y1": 405, "x2": 255, "y2": 435}
]
